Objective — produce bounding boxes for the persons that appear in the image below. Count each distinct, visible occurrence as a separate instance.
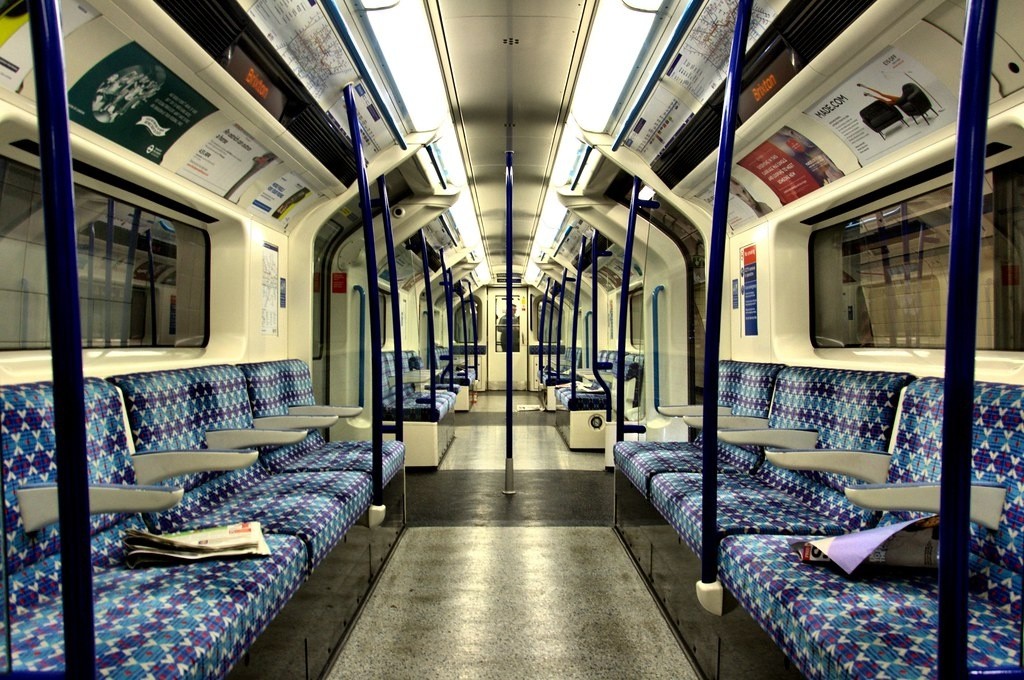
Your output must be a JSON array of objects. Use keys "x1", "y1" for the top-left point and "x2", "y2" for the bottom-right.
[{"x1": 497, "y1": 304, "x2": 519, "y2": 352}]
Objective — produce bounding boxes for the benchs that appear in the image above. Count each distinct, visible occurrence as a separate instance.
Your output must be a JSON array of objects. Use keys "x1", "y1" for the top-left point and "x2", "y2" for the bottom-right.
[
  {"x1": 0, "y1": 346, "x2": 477, "y2": 680},
  {"x1": 537, "y1": 347, "x2": 1024, "y2": 680}
]
